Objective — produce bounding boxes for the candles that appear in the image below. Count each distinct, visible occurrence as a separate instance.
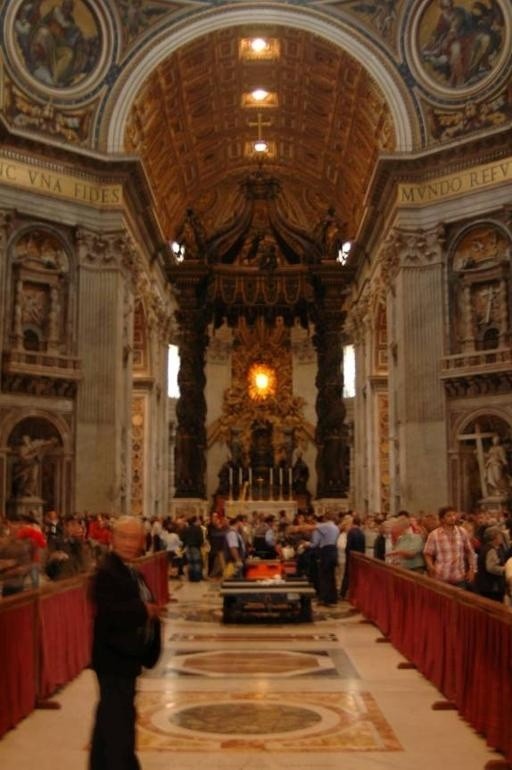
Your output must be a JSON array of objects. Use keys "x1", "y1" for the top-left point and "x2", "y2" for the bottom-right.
[
  {"x1": 229, "y1": 466, "x2": 253, "y2": 485},
  {"x1": 269, "y1": 467, "x2": 293, "y2": 485}
]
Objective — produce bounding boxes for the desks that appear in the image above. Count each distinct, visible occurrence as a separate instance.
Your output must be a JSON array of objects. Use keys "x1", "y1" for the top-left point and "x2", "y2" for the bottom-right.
[{"x1": 221, "y1": 567, "x2": 319, "y2": 621}]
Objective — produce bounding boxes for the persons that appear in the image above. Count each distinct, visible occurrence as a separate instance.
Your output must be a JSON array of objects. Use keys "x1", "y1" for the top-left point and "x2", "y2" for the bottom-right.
[
  {"x1": 420, "y1": 0, "x2": 507, "y2": 90},
  {"x1": 364, "y1": 507, "x2": 511, "y2": 604},
  {"x1": 2, "y1": 509, "x2": 115, "y2": 598},
  {"x1": 16, "y1": 435, "x2": 54, "y2": 497},
  {"x1": 81, "y1": 516, "x2": 170, "y2": 770},
  {"x1": 204, "y1": 392, "x2": 319, "y2": 497},
  {"x1": 12, "y1": 1, "x2": 87, "y2": 88},
  {"x1": 474, "y1": 434, "x2": 511, "y2": 499},
  {"x1": 142, "y1": 508, "x2": 367, "y2": 607}
]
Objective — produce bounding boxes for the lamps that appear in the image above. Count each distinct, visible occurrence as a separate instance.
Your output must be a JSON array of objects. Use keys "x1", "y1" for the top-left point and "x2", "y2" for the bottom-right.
[
  {"x1": 172, "y1": 236, "x2": 187, "y2": 262},
  {"x1": 335, "y1": 238, "x2": 352, "y2": 267}
]
